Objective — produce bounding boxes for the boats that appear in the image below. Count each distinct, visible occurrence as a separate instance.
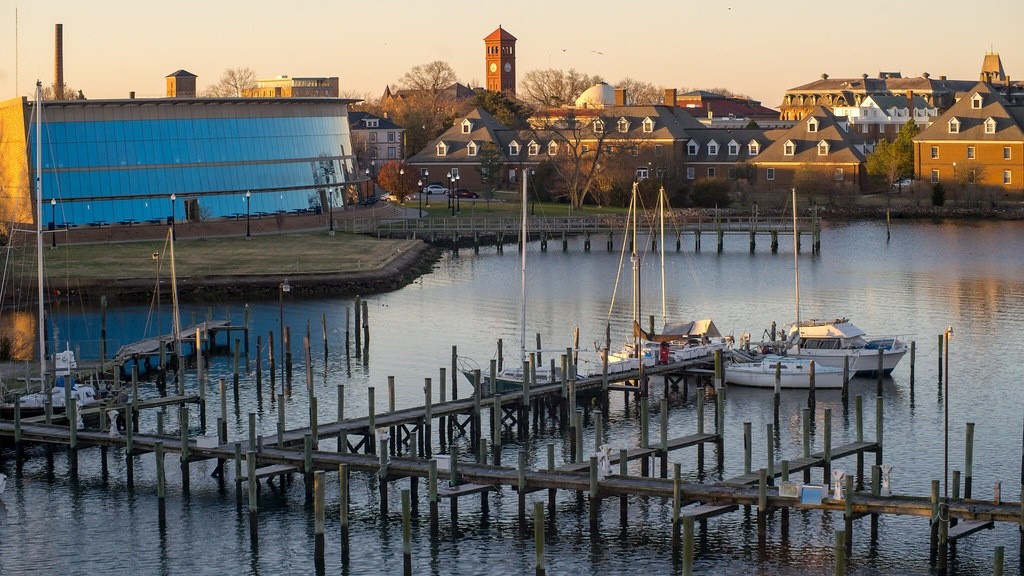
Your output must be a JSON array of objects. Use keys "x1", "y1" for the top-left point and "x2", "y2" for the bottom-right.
[{"x1": 723, "y1": 350, "x2": 858, "y2": 389}]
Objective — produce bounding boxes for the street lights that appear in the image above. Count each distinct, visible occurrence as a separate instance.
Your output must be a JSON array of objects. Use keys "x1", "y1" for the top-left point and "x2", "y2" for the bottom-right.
[
  {"x1": 456, "y1": 175, "x2": 460, "y2": 212},
  {"x1": 245, "y1": 191, "x2": 251, "y2": 237},
  {"x1": 400, "y1": 169, "x2": 404, "y2": 203},
  {"x1": 424, "y1": 170, "x2": 429, "y2": 206},
  {"x1": 171, "y1": 193, "x2": 176, "y2": 242},
  {"x1": 51, "y1": 198, "x2": 57, "y2": 247},
  {"x1": 365, "y1": 169, "x2": 369, "y2": 200},
  {"x1": 371, "y1": 162, "x2": 375, "y2": 195},
  {"x1": 451, "y1": 176, "x2": 457, "y2": 216},
  {"x1": 447, "y1": 172, "x2": 452, "y2": 210},
  {"x1": 417, "y1": 181, "x2": 422, "y2": 218},
  {"x1": 329, "y1": 187, "x2": 333, "y2": 227}
]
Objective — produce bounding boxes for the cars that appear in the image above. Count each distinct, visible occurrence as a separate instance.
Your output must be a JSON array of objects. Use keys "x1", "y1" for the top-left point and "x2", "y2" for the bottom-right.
[{"x1": 380, "y1": 184, "x2": 480, "y2": 204}]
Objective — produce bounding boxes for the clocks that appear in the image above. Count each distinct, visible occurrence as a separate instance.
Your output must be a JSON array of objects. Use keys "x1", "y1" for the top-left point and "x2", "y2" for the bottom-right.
[
  {"x1": 490, "y1": 63, "x2": 497, "y2": 73},
  {"x1": 504, "y1": 63, "x2": 512, "y2": 73}
]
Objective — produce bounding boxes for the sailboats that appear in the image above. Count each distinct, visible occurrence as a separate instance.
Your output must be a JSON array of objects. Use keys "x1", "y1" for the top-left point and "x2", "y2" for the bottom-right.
[
  {"x1": 593, "y1": 180, "x2": 730, "y2": 376},
  {"x1": 461, "y1": 168, "x2": 611, "y2": 401},
  {"x1": 734, "y1": 186, "x2": 911, "y2": 379},
  {"x1": 2, "y1": 82, "x2": 129, "y2": 431}
]
